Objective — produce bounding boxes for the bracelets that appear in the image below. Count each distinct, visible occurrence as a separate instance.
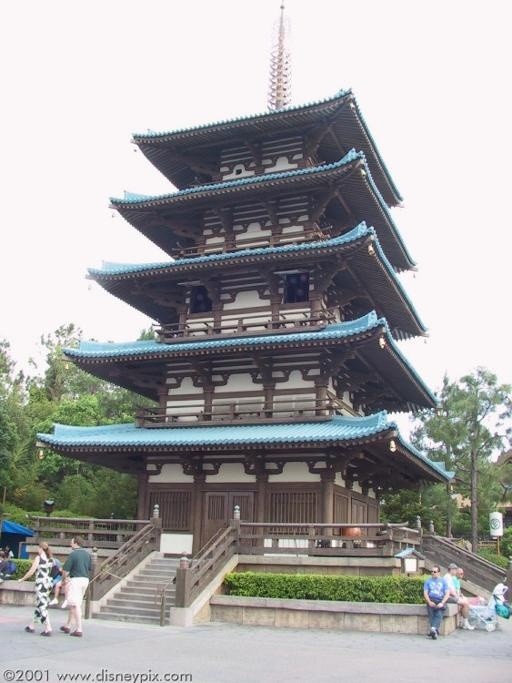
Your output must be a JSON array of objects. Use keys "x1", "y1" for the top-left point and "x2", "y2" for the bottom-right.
[
  {"x1": 21, "y1": 577, "x2": 25, "y2": 579},
  {"x1": 441, "y1": 599, "x2": 445, "y2": 603}
]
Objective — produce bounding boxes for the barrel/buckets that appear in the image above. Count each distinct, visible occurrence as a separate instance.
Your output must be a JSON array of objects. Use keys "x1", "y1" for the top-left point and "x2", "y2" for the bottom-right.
[{"x1": 342, "y1": 527, "x2": 361, "y2": 537}]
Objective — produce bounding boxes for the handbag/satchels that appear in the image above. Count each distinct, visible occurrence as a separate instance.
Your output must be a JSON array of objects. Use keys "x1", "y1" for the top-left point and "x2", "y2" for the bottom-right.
[
  {"x1": 494, "y1": 595, "x2": 512, "y2": 619},
  {"x1": 49, "y1": 565, "x2": 62, "y2": 579}
]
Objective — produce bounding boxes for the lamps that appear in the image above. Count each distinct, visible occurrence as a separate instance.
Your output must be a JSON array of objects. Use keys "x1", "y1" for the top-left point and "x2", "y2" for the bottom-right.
[{"x1": 387, "y1": 438, "x2": 398, "y2": 453}]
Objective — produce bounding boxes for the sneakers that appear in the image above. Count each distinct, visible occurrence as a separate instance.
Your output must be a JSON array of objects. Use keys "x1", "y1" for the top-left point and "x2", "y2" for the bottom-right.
[
  {"x1": 458, "y1": 622, "x2": 475, "y2": 630},
  {"x1": 425, "y1": 627, "x2": 439, "y2": 640},
  {"x1": 24, "y1": 626, "x2": 84, "y2": 637},
  {"x1": 49, "y1": 598, "x2": 67, "y2": 609}
]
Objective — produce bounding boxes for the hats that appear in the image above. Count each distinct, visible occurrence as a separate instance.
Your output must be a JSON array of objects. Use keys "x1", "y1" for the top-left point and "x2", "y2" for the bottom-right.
[{"x1": 447, "y1": 562, "x2": 458, "y2": 569}]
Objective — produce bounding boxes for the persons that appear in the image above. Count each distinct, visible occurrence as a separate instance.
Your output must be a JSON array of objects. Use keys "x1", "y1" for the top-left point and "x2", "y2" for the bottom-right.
[
  {"x1": 58, "y1": 535, "x2": 92, "y2": 638},
  {"x1": 51, "y1": 557, "x2": 62, "y2": 589},
  {"x1": 16, "y1": 539, "x2": 59, "y2": 637},
  {"x1": 423, "y1": 564, "x2": 452, "y2": 640},
  {"x1": 452, "y1": 566, "x2": 468, "y2": 599},
  {"x1": 443, "y1": 561, "x2": 477, "y2": 630},
  {"x1": 0, "y1": 542, "x2": 16, "y2": 579},
  {"x1": 47, "y1": 566, "x2": 70, "y2": 608}
]
